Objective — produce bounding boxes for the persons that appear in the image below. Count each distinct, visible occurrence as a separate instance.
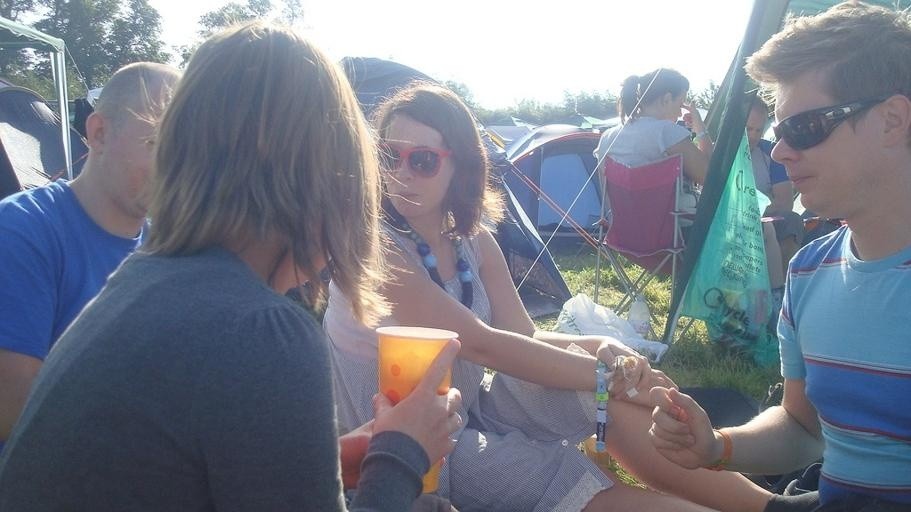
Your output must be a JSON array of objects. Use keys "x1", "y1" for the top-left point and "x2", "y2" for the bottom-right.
[
  {"x1": 0, "y1": 61, "x2": 181, "y2": 446},
  {"x1": 592, "y1": 68, "x2": 715, "y2": 245},
  {"x1": 321, "y1": 78, "x2": 820, "y2": 512},
  {"x1": 0, "y1": 22, "x2": 463, "y2": 512},
  {"x1": 746, "y1": 95, "x2": 806, "y2": 324},
  {"x1": 645, "y1": 0, "x2": 910, "y2": 512}
]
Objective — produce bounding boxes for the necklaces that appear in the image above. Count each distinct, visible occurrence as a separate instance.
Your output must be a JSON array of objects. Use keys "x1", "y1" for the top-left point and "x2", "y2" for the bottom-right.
[{"x1": 380, "y1": 196, "x2": 474, "y2": 309}]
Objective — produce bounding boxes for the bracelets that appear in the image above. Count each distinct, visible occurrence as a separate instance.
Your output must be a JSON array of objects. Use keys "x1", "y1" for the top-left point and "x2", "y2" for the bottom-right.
[{"x1": 704, "y1": 428, "x2": 732, "y2": 471}]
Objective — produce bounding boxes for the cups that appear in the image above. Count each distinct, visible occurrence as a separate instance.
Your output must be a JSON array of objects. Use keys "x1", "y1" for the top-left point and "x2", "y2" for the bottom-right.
[{"x1": 374, "y1": 325, "x2": 459, "y2": 493}]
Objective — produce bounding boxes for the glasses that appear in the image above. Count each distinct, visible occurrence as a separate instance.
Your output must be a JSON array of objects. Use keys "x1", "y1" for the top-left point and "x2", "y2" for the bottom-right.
[
  {"x1": 771, "y1": 96, "x2": 882, "y2": 152},
  {"x1": 376, "y1": 143, "x2": 452, "y2": 177}
]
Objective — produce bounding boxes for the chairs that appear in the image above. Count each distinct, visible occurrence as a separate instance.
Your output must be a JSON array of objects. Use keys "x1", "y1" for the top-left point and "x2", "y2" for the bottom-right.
[{"x1": 596, "y1": 152, "x2": 698, "y2": 322}]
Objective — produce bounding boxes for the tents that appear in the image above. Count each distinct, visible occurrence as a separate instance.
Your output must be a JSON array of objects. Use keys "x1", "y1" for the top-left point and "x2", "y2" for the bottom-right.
[
  {"x1": 484, "y1": 106, "x2": 708, "y2": 255},
  {"x1": 660, "y1": 0, "x2": 910, "y2": 372},
  {"x1": 0, "y1": 78, "x2": 89, "y2": 201},
  {"x1": 339, "y1": 55, "x2": 573, "y2": 321}
]
